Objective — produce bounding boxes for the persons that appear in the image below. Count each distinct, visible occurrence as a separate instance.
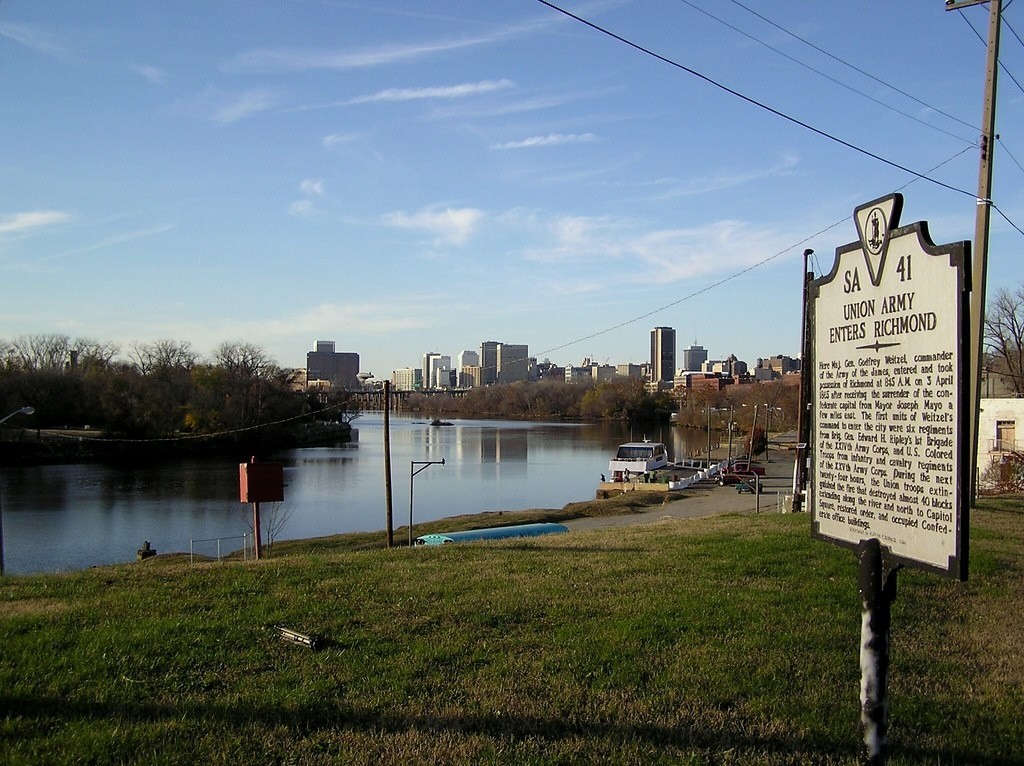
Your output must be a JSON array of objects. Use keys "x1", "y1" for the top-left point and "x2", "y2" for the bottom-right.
[
  {"x1": 601, "y1": 474, "x2": 606, "y2": 481},
  {"x1": 624, "y1": 468, "x2": 630, "y2": 481}
]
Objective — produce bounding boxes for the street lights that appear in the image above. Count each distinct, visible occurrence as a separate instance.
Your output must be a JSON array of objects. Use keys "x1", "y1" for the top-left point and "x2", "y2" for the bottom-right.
[{"x1": 0, "y1": 406, "x2": 37, "y2": 578}]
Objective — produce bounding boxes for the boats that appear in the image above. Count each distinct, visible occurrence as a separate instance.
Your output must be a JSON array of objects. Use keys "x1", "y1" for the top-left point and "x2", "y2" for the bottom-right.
[
  {"x1": 736, "y1": 481, "x2": 764, "y2": 493},
  {"x1": 609, "y1": 433, "x2": 668, "y2": 484},
  {"x1": 430, "y1": 420, "x2": 455, "y2": 426}
]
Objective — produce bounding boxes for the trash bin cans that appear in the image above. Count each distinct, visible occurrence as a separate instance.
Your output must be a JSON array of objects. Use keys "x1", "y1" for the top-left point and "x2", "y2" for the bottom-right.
[
  {"x1": 641, "y1": 470, "x2": 650, "y2": 483},
  {"x1": 774, "y1": 488, "x2": 793, "y2": 513}
]
